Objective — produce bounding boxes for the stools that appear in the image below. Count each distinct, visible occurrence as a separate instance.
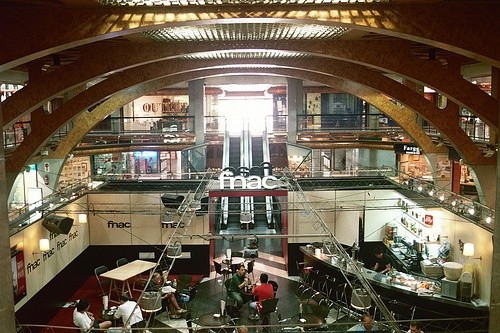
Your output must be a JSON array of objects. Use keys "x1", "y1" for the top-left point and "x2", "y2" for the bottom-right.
[{"x1": 345, "y1": 302, "x2": 371, "y2": 327}]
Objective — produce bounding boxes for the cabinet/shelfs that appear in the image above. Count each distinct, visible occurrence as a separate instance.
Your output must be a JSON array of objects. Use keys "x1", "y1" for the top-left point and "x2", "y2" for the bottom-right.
[{"x1": 299, "y1": 235, "x2": 489, "y2": 333}]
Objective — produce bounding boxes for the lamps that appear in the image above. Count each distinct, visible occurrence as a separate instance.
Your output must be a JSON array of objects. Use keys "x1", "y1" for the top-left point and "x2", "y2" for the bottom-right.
[
  {"x1": 32, "y1": 239, "x2": 50, "y2": 257},
  {"x1": 462, "y1": 243, "x2": 482, "y2": 262},
  {"x1": 72, "y1": 212, "x2": 88, "y2": 227}
]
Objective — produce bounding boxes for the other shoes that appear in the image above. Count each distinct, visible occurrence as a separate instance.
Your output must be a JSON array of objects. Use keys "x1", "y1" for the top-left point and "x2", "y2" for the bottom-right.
[
  {"x1": 176, "y1": 308, "x2": 188, "y2": 314},
  {"x1": 255, "y1": 315, "x2": 259, "y2": 320},
  {"x1": 170, "y1": 314, "x2": 182, "y2": 319},
  {"x1": 249, "y1": 314, "x2": 254, "y2": 320}
]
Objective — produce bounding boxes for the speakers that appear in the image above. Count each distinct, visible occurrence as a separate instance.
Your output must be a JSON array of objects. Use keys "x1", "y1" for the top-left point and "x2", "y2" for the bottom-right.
[
  {"x1": 42, "y1": 215, "x2": 74, "y2": 235},
  {"x1": 161, "y1": 194, "x2": 184, "y2": 209}
]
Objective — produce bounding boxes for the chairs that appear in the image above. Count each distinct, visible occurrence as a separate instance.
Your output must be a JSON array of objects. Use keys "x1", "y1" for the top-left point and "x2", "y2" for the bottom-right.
[
  {"x1": 154, "y1": 292, "x2": 186, "y2": 319},
  {"x1": 138, "y1": 253, "x2": 155, "y2": 283},
  {"x1": 185, "y1": 305, "x2": 239, "y2": 333},
  {"x1": 213, "y1": 251, "x2": 256, "y2": 286},
  {"x1": 95, "y1": 266, "x2": 119, "y2": 299},
  {"x1": 129, "y1": 317, "x2": 147, "y2": 333},
  {"x1": 224, "y1": 278, "x2": 279, "y2": 326},
  {"x1": 117, "y1": 258, "x2": 134, "y2": 287}
]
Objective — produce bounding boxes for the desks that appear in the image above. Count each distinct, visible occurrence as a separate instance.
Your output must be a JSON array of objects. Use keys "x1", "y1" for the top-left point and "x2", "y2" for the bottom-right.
[
  {"x1": 240, "y1": 283, "x2": 262, "y2": 316},
  {"x1": 100, "y1": 260, "x2": 160, "y2": 305},
  {"x1": 222, "y1": 256, "x2": 246, "y2": 279},
  {"x1": 141, "y1": 305, "x2": 162, "y2": 326},
  {"x1": 200, "y1": 313, "x2": 232, "y2": 333},
  {"x1": 99, "y1": 306, "x2": 121, "y2": 327}
]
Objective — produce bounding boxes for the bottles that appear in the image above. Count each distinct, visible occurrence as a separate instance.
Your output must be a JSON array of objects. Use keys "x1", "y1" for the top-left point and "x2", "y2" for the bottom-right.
[
  {"x1": 172, "y1": 279, "x2": 178, "y2": 292},
  {"x1": 244, "y1": 280, "x2": 248, "y2": 294},
  {"x1": 247, "y1": 276, "x2": 252, "y2": 293}
]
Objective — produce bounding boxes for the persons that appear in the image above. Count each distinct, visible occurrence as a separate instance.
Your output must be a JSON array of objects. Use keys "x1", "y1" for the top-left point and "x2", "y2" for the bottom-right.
[
  {"x1": 238, "y1": 325, "x2": 248, "y2": 333},
  {"x1": 227, "y1": 265, "x2": 251, "y2": 311},
  {"x1": 305, "y1": 107, "x2": 399, "y2": 128},
  {"x1": 346, "y1": 313, "x2": 379, "y2": 331},
  {"x1": 95, "y1": 157, "x2": 152, "y2": 173},
  {"x1": 249, "y1": 273, "x2": 274, "y2": 321},
  {"x1": 73, "y1": 297, "x2": 112, "y2": 333},
  {"x1": 112, "y1": 293, "x2": 147, "y2": 333},
  {"x1": 144, "y1": 272, "x2": 188, "y2": 319},
  {"x1": 372, "y1": 245, "x2": 392, "y2": 274},
  {"x1": 134, "y1": 118, "x2": 190, "y2": 130},
  {"x1": 406, "y1": 319, "x2": 424, "y2": 333}
]
